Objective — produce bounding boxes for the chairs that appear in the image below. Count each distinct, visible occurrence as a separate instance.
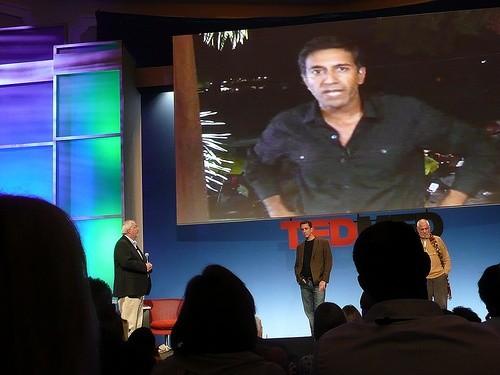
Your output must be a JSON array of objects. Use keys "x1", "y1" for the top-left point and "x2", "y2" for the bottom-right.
[{"x1": 142, "y1": 299, "x2": 185, "y2": 352}]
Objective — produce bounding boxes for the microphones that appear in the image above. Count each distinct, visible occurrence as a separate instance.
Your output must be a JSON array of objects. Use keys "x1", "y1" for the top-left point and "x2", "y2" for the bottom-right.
[{"x1": 145, "y1": 253, "x2": 149, "y2": 263}]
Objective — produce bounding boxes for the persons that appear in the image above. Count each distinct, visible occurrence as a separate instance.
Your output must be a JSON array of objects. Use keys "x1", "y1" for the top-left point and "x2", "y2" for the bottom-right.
[
  {"x1": 452, "y1": 305, "x2": 482, "y2": 323},
  {"x1": 310, "y1": 218, "x2": 500, "y2": 375},
  {"x1": 478, "y1": 263, "x2": 500, "y2": 334},
  {"x1": 112, "y1": 219, "x2": 154, "y2": 338},
  {"x1": 295, "y1": 221, "x2": 332, "y2": 342},
  {"x1": 417, "y1": 218, "x2": 452, "y2": 309},
  {"x1": 88, "y1": 263, "x2": 361, "y2": 375},
  {"x1": 0, "y1": 193, "x2": 100, "y2": 375},
  {"x1": 245, "y1": 36, "x2": 500, "y2": 219}
]
